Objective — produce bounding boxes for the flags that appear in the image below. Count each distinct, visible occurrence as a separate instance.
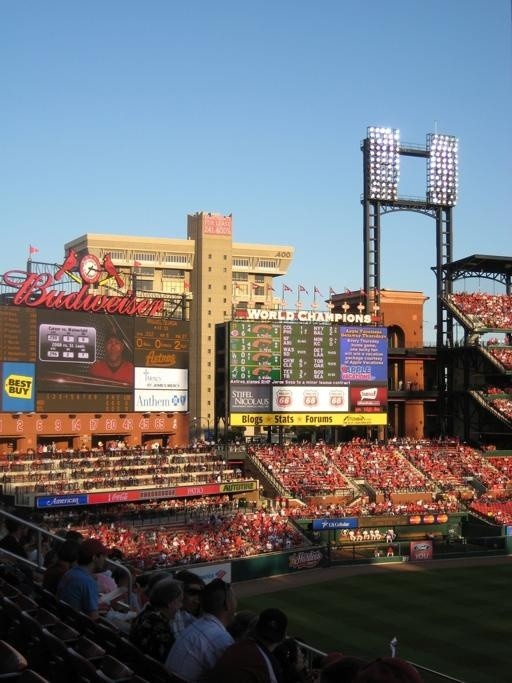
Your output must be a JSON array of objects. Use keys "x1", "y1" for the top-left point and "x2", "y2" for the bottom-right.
[{"x1": 234, "y1": 282, "x2": 381, "y2": 297}]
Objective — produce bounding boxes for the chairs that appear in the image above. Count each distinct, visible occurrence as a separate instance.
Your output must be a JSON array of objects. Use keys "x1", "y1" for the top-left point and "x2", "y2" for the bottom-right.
[{"x1": 0, "y1": 575, "x2": 187, "y2": 682}]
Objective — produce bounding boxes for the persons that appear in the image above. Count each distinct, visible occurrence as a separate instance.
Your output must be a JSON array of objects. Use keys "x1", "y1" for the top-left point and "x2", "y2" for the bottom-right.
[
  {"x1": 2, "y1": 435, "x2": 304, "y2": 575},
  {"x1": 484, "y1": 386, "x2": 512, "y2": 421},
  {"x1": 450, "y1": 291, "x2": 512, "y2": 328},
  {"x1": 227, "y1": 436, "x2": 512, "y2": 558},
  {"x1": 2, "y1": 508, "x2": 421, "y2": 682},
  {"x1": 486, "y1": 335, "x2": 512, "y2": 371},
  {"x1": 87, "y1": 332, "x2": 134, "y2": 386}
]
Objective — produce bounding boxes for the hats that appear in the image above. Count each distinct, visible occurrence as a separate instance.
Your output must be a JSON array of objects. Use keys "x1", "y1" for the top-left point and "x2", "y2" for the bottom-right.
[
  {"x1": 353, "y1": 655, "x2": 424, "y2": 683},
  {"x1": 78, "y1": 537, "x2": 115, "y2": 558}
]
